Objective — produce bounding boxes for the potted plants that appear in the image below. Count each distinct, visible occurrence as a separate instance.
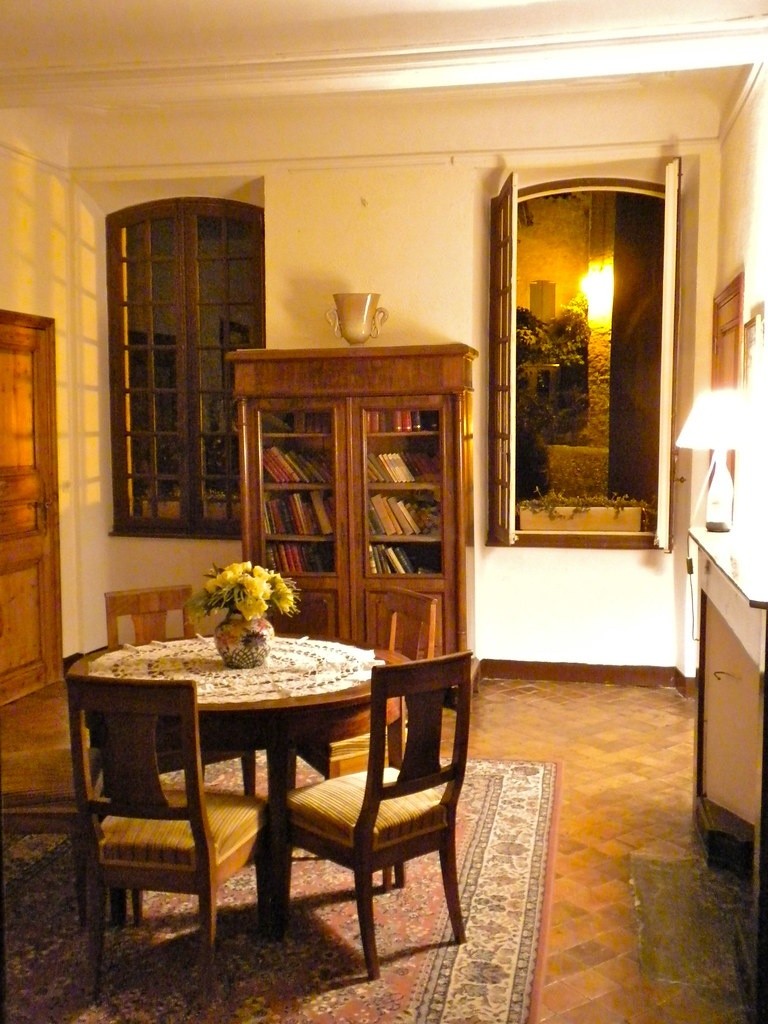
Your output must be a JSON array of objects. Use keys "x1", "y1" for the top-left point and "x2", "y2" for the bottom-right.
[{"x1": 515, "y1": 485, "x2": 657, "y2": 532}]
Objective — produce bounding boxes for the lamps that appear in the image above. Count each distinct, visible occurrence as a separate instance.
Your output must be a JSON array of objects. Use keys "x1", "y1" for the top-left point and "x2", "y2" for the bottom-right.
[{"x1": 675, "y1": 391, "x2": 754, "y2": 532}]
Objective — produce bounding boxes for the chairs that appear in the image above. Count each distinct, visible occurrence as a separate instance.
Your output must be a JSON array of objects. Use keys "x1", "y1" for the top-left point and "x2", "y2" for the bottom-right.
[{"x1": 66, "y1": 584, "x2": 474, "y2": 1002}]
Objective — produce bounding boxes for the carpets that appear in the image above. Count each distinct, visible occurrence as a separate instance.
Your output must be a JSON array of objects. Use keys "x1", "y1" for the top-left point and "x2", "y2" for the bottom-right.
[{"x1": 0, "y1": 749, "x2": 563, "y2": 1024}]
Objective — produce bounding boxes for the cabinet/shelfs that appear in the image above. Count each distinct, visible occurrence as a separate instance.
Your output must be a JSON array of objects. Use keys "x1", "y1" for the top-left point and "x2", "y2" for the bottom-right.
[{"x1": 225, "y1": 342, "x2": 481, "y2": 706}]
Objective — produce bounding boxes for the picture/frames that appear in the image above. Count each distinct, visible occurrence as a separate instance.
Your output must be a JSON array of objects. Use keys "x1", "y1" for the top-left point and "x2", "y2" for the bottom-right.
[{"x1": 743, "y1": 314, "x2": 762, "y2": 389}]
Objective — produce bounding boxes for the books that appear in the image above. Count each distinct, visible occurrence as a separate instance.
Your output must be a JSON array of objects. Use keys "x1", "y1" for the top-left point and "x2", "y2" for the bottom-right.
[
  {"x1": 367, "y1": 411, "x2": 438, "y2": 433},
  {"x1": 263, "y1": 447, "x2": 334, "y2": 484},
  {"x1": 262, "y1": 412, "x2": 331, "y2": 433},
  {"x1": 266, "y1": 544, "x2": 334, "y2": 573},
  {"x1": 367, "y1": 451, "x2": 442, "y2": 483},
  {"x1": 368, "y1": 494, "x2": 441, "y2": 536},
  {"x1": 262, "y1": 490, "x2": 335, "y2": 536},
  {"x1": 368, "y1": 543, "x2": 441, "y2": 575}
]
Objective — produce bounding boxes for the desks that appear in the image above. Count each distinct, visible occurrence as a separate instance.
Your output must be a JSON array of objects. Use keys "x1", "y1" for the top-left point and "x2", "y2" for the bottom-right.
[
  {"x1": 688, "y1": 525, "x2": 768, "y2": 1024},
  {"x1": 67, "y1": 634, "x2": 412, "y2": 936}
]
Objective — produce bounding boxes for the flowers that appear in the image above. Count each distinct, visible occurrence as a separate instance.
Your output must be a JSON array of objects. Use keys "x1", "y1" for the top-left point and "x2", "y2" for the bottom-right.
[{"x1": 185, "y1": 561, "x2": 300, "y2": 621}]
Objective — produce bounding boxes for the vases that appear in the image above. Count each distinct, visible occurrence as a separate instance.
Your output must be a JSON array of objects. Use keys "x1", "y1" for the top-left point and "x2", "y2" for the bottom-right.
[{"x1": 214, "y1": 610, "x2": 275, "y2": 669}]
